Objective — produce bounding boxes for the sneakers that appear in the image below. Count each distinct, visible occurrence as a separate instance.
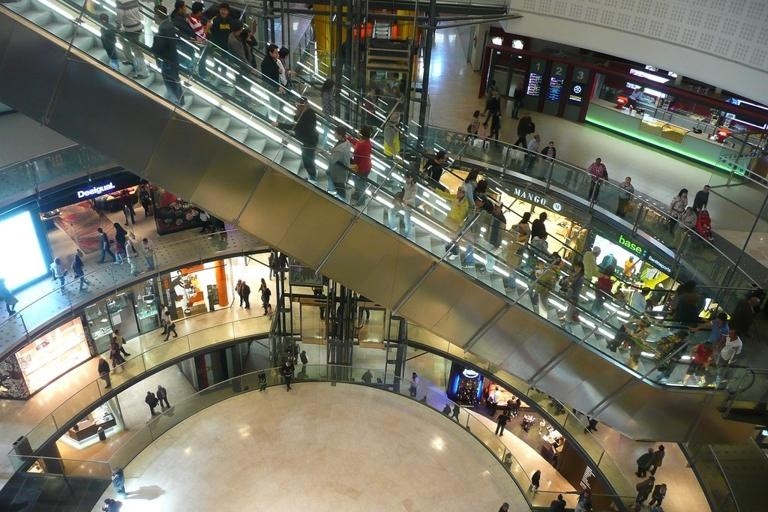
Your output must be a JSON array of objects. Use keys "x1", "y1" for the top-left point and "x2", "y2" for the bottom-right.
[
  {"x1": 448, "y1": 251, "x2": 552, "y2": 313},
  {"x1": 121, "y1": 59, "x2": 253, "y2": 108},
  {"x1": 558, "y1": 316, "x2": 718, "y2": 389},
  {"x1": 301, "y1": 176, "x2": 367, "y2": 208}
]
